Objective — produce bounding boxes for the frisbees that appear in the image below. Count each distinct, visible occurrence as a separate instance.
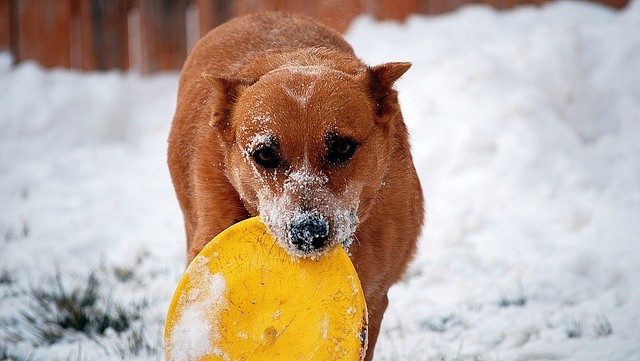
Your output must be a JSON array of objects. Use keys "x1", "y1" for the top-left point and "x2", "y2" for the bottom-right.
[{"x1": 163, "y1": 215, "x2": 369, "y2": 361}]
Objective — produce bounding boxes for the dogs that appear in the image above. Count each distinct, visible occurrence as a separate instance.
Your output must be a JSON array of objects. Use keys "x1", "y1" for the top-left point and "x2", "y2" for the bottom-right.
[{"x1": 166, "y1": 9, "x2": 425, "y2": 361}]
